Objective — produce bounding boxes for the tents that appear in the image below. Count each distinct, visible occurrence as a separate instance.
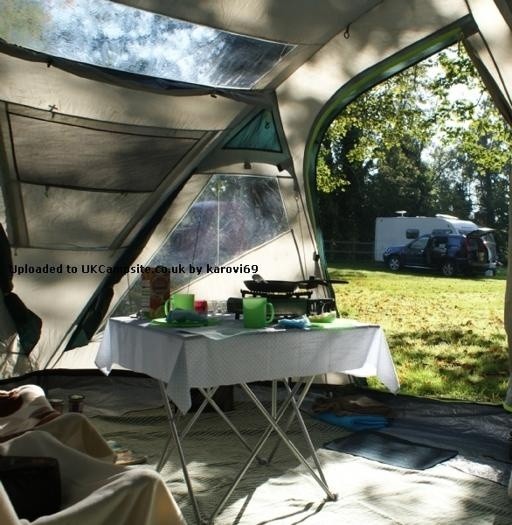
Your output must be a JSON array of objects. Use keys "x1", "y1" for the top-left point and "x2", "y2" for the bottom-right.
[{"x1": 1, "y1": 0, "x2": 510, "y2": 525}]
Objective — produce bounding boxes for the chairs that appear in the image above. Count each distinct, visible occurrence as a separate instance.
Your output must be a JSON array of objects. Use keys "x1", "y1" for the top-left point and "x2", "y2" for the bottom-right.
[{"x1": 0, "y1": 384, "x2": 186, "y2": 524}]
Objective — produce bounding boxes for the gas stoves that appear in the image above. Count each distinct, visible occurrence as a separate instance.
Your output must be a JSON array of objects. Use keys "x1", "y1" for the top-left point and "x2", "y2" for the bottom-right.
[{"x1": 226, "y1": 289, "x2": 336, "y2": 324}]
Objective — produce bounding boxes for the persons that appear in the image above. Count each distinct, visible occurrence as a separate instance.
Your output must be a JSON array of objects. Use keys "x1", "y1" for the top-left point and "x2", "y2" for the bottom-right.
[
  {"x1": 0, "y1": 380, "x2": 60, "y2": 444},
  {"x1": 1, "y1": 411, "x2": 187, "y2": 524}
]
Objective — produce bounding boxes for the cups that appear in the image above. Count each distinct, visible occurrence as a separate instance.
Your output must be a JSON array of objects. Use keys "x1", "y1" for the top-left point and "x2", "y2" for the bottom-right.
[
  {"x1": 48, "y1": 398, "x2": 64, "y2": 414},
  {"x1": 68, "y1": 393, "x2": 85, "y2": 415},
  {"x1": 242, "y1": 296, "x2": 275, "y2": 330},
  {"x1": 164, "y1": 292, "x2": 195, "y2": 321},
  {"x1": 194, "y1": 300, "x2": 208, "y2": 318}
]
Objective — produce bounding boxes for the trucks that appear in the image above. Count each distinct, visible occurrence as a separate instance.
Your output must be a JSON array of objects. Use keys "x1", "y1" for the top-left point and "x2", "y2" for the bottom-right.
[{"x1": 369, "y1": 211, "x2": 482, "y2": 265}]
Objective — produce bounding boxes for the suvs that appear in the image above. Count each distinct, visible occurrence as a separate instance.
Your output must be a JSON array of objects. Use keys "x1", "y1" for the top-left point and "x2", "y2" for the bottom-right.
[{"x1": 379, "y1": 222, "x2": 502, "y2": 282}]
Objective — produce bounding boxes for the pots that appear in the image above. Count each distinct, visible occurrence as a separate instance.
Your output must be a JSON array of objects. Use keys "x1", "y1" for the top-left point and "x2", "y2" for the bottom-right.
[{"x1": 243, "y1": 274, "x2": 350, "y2": 294}]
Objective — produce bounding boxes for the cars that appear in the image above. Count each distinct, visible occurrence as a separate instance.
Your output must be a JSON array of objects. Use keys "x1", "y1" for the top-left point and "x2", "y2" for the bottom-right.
[{"x1": 166, "y1": 194, "x2": 251, "y2": 273}]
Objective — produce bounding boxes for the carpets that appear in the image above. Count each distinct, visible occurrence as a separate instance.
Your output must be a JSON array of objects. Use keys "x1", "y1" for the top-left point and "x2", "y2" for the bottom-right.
[{"x1": 323, "y1": 428, "x2": 458, "y2": 471}]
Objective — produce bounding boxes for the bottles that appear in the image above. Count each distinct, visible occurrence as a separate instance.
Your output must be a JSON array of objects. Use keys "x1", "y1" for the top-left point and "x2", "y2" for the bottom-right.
[{"x1": 141, "y1": 279, "x2": 152, "y2": 322}]
